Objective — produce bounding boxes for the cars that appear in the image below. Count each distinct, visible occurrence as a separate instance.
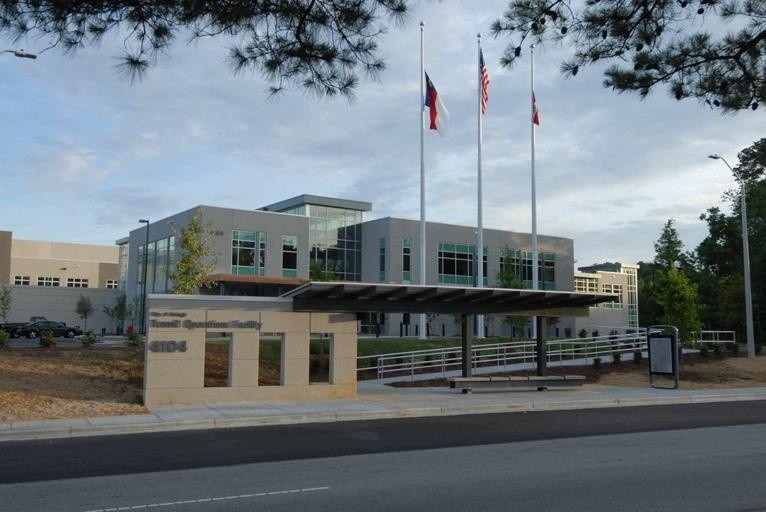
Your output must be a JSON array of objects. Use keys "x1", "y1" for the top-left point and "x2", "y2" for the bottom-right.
[{"x1": 19, "y1": 320, "x2": 79, "y2": 339}]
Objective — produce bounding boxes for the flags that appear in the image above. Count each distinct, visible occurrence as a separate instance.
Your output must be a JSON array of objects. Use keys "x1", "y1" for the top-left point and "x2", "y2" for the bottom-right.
[
  {"x1": 481, "y1": 48, "x2": 490, "y2": 114},
  {"x1": 532, "y1": 93, "x2": 540, "y2": 127},
  {"x1": 424, "y1": 72, "x2": 449, "y2": 137}
]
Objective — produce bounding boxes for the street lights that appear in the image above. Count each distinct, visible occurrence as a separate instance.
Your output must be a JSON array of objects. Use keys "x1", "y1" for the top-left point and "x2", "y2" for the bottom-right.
[
  {"x1": 139, "y1": 219, "x2": 148, "y2": 335},
  {"x1": 707, "y1": 155, "x2": 755, "y2": 358}
]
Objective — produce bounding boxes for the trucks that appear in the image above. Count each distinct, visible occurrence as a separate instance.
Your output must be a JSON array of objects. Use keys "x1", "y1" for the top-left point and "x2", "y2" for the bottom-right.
[{"x1": 0, "y1": 315, "x2": 47, "y2": 339}]
